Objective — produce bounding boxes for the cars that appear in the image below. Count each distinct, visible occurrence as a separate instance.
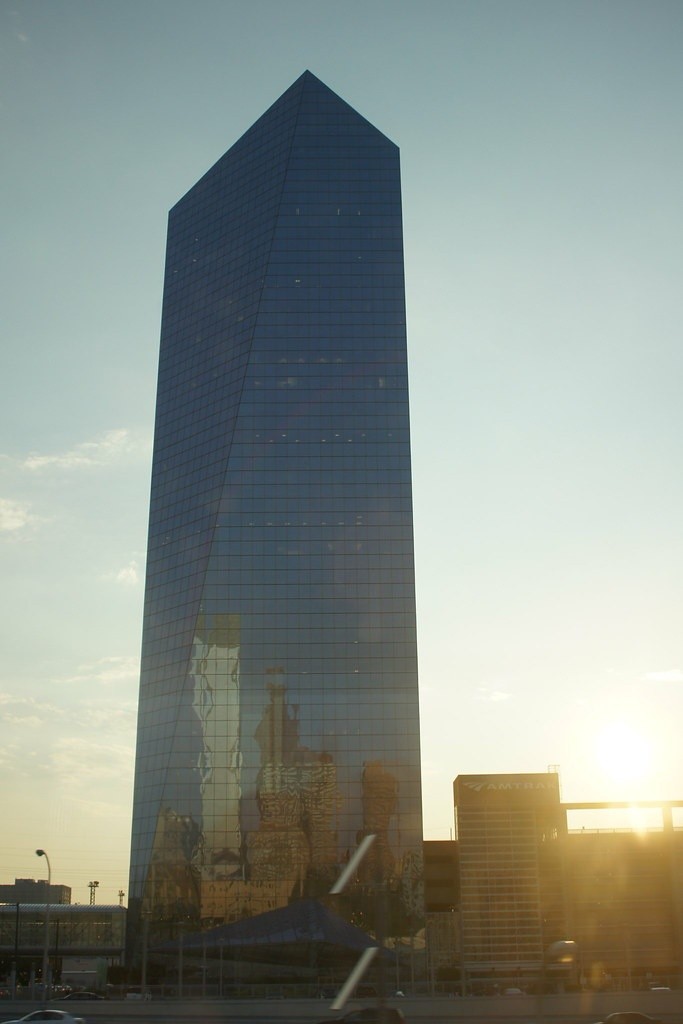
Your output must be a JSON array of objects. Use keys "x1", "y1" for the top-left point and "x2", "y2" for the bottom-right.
[
  {"x1": 0, "y1": 1009, "x2": 84, "y2": 1024},
  {"x1": 260, "y1": 980, "x2": 671, "y2": 999},
  {"x1": 0, "y1": 981, "x2": 86, "y2": 999},
  {"x1": 49, "y1": 991, "x2": 106, "y2": 1001},
  {"x1": 123, "y1": 987, "x2": 154, "y2": 1001},
  {"x1": 593, "y1": 1011, "x2": 663, "y2": 1024}
]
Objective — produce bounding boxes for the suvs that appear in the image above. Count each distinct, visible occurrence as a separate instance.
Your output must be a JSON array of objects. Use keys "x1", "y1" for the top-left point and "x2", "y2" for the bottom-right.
[{"x1": 319, "y1": 1006, "x2": 405, "y2": 1024}]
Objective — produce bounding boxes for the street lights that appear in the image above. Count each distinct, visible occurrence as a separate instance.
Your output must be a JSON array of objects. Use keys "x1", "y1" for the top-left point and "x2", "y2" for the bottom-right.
[{"x1": 37, "y1": 849, "x2": 51, "y2": 1001}]
